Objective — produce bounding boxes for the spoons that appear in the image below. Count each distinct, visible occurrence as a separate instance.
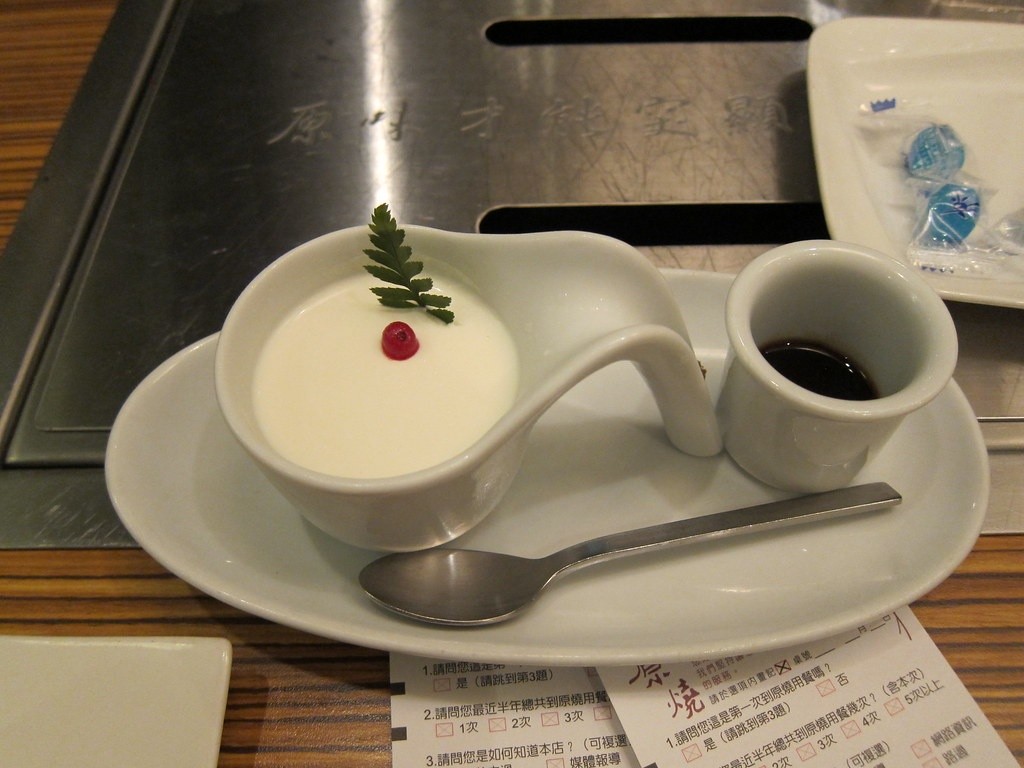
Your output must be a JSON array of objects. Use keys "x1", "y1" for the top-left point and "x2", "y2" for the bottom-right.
[{"x1": 356, "y1": 482, "x2": 899, "y2": 627}]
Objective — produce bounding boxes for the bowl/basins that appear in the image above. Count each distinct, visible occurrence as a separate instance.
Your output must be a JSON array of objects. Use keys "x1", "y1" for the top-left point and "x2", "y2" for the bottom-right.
[{"x1": 213, "y1": 219, "x2": 724, "y2": 550}]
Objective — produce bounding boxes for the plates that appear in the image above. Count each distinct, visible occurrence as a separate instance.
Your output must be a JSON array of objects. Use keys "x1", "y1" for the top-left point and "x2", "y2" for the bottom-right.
[
  {"x1": 100, "y1": 269, "x2": 991, "y2": 668},
  {"x1": 0, "y1": 636, "x2": 233, "y2": 768},
  {"x1": 804, "y1": 13, "x2": 1024, "y2": 318}
]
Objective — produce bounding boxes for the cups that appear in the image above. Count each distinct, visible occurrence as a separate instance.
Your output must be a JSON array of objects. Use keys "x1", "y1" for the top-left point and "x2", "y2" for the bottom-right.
[{"x1": 717, "y1": 241, "x2": 958, "y2": 492}]
